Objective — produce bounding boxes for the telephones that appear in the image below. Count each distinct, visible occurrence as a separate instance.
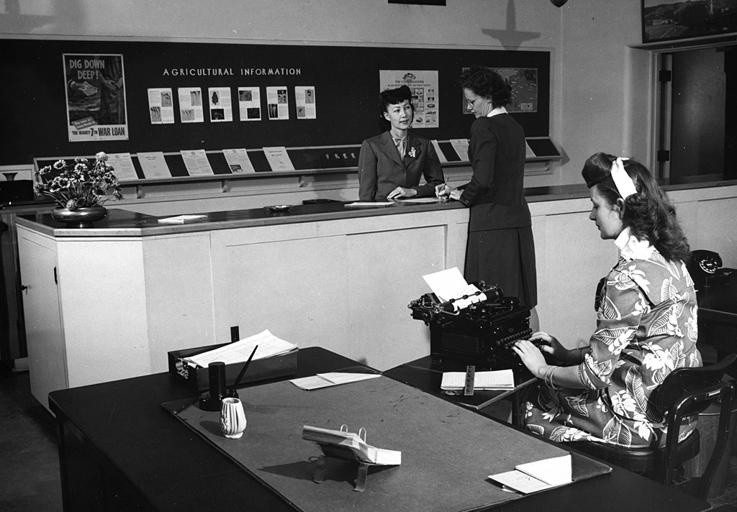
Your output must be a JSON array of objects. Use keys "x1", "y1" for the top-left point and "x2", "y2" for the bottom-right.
[{"x1": 686, "y1": 249, "x2": 729, "y2": 291}]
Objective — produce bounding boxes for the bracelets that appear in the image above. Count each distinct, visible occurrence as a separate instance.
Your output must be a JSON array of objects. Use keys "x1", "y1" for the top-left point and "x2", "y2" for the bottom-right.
[
  {"x1": 575, "y1": 338, "x2": 589, "y2": 358},
  {"x1": 539, "y1": 365, "x2": 564, "y2": 392}
]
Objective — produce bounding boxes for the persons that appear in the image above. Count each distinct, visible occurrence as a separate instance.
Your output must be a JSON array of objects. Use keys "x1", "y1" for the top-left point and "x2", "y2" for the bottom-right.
[
  {"x1": 511, "y1": 152, "x2": 703, "y2": 449},
  {"x1": 358, "y1": 84, "x2": 445, "y2": 200},
  {"x1": 99, "y1": 55, "x2": 126, "y2": 125},
  {"x1": 434, "y1": 66, "x2": 539, "y2": 331}
]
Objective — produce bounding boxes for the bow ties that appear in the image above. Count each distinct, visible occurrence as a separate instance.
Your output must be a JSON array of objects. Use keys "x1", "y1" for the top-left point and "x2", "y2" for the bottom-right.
[{"x1": 393, "y1": 137, "x2": 407, "y2": 147}]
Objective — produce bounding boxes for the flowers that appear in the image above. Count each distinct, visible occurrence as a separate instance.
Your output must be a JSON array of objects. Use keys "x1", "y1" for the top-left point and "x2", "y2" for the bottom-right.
[{"x1": 33, "y1": 152, "x2": 123, "y2": 209}]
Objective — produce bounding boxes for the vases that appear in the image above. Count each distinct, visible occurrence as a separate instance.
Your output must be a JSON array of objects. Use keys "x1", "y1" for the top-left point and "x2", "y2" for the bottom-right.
[{"x1": 51, "y1": 204, "x2": 108, "y2": 229}]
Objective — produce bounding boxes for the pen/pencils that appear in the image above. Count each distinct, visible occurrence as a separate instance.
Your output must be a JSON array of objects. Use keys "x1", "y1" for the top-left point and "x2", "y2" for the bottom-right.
[
  {"x1": 438, "y1": 177, "x2": 449, "y2": 200},
  {"x1": 233, "y1": 343, "x2": 259, "y2": 387}
]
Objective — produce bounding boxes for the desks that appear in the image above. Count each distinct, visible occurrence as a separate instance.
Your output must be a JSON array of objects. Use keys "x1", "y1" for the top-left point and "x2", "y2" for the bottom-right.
[
  {"x1": 49, "y1": 349, "x2": 713, "y2": 512},
  {"x1": 384, "y1": 354, "x2": 573, "y2": 429}
]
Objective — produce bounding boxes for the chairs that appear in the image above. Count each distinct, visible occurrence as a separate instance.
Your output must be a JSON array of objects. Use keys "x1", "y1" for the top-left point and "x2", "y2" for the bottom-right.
[{"x1": 644, "y1": 353, "x2": 737, "y2": 512}]
